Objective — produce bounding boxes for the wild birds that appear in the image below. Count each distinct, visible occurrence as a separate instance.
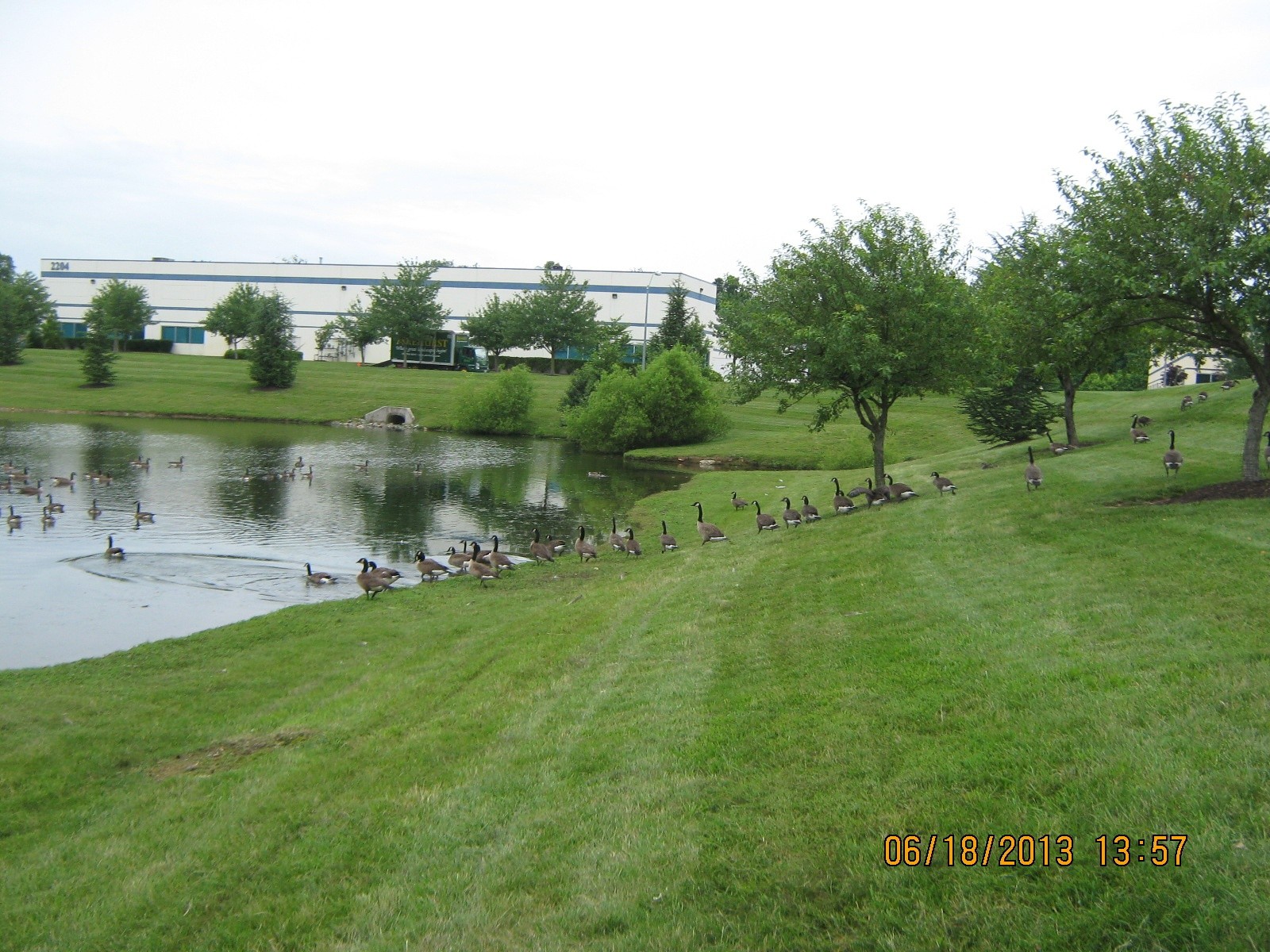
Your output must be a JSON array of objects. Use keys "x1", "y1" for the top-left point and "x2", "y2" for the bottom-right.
[
  {"x1": 1, "y1": 456, "x2": 959, "y2": 601},
  {"x1": 1162, "y1": 430, "x2": 1183, "y2": 479},
  {"x1": 1044, "y1": 430, "x2": 1069, "y2": 457},
  {"x1": 1130, "y1": 414, "x2": 1155, "y2": 444},
  {"x1": 1221, "y1": 374, "x2": 1257, "y2": 392},
  {"x1": 1025, "y1": 445, "x2": 1043, "y2": 492},
  {"x1": 1181, "y1": 395, "x2": 1194, "y2": 411},
  {"x1": 1262, "y1": 431, "x2": 1270, "y2": 470},
  {"x1": 1197, "y1": 392, "x2": 1208, "y2": 405}
]
